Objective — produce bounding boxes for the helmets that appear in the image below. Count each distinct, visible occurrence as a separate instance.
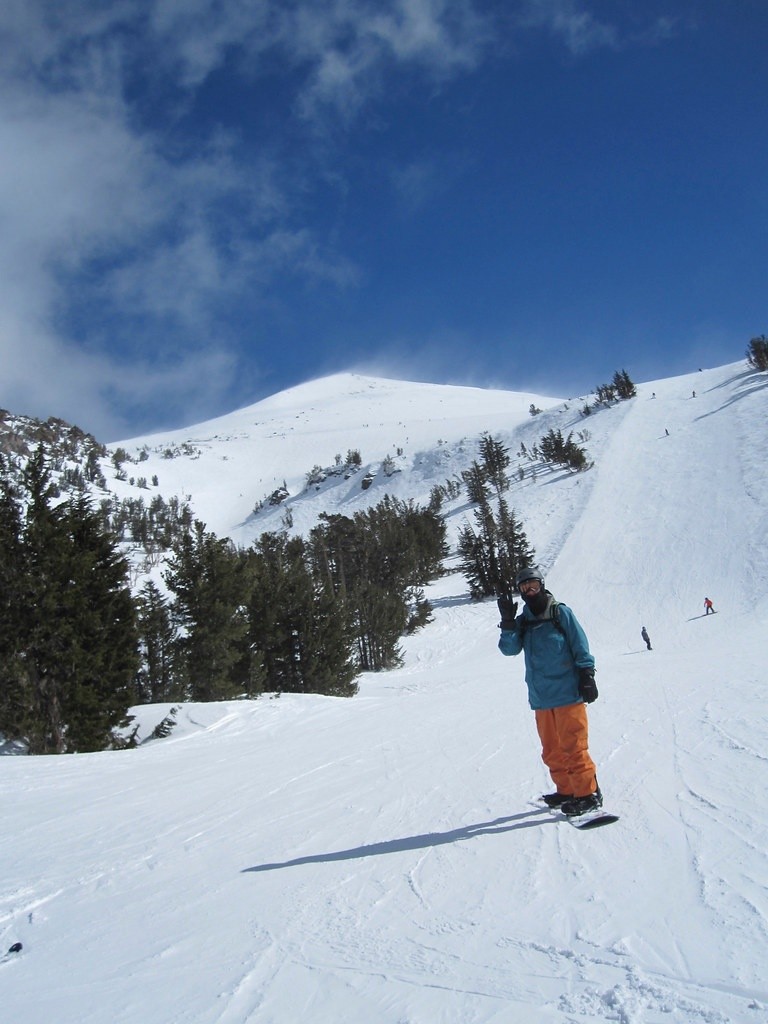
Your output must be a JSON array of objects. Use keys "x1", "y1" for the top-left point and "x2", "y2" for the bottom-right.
[{"x1": 516, "y1": 568, "x2": 544, "y2": 591}]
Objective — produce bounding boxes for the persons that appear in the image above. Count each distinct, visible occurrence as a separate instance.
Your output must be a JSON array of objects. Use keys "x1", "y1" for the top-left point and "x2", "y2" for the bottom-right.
[
  {"x1": 704, "y1": 598, "x2": 714, "y2": 615},
  {"x1": 641, "y1": 627, "x2": 652, "y2": 651},
  {"x1": 497, "y1": 568, "x2": 603, "y2": 815}
]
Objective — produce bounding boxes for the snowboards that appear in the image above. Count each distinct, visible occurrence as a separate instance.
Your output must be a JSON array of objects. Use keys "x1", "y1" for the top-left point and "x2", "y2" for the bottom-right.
[{"x1": 541, "y1": 792, "x2": 620, "y2": 829}]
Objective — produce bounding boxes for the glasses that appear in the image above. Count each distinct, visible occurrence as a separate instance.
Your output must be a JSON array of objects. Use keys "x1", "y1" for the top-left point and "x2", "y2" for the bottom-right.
[{"x1": 519, "y1": 579, "x2": 544, "y2": 593}]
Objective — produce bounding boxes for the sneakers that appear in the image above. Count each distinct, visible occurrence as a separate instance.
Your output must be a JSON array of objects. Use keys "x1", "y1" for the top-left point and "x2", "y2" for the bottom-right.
[
  {"x1": 544, "y1": 793, "x2": 573, "y2": 808},
  {"x1": 561, "y1": 792, "x2": 603, "y2": 816}
]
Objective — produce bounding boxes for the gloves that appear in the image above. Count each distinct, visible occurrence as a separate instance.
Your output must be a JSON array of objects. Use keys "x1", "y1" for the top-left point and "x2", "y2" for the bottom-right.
[
  {"x1": 578, "y1": 675, "x2": 598, "y2": 704},
  {"x1": 497, "y1": 591, "x2": 518, "y2": 629}
]
